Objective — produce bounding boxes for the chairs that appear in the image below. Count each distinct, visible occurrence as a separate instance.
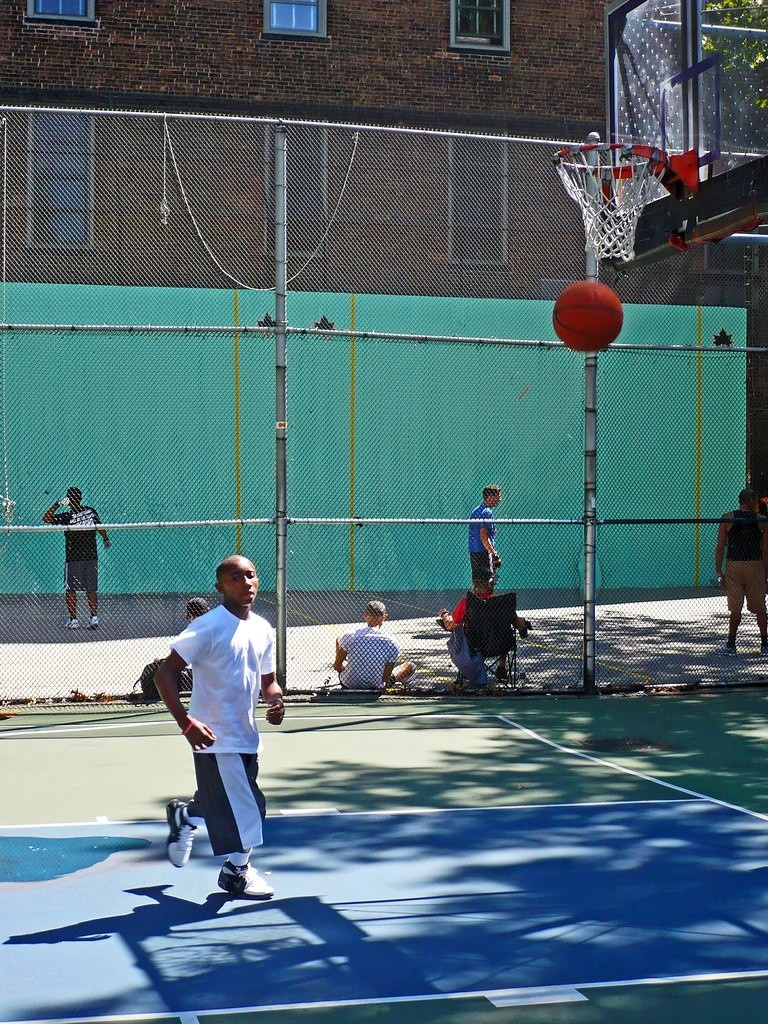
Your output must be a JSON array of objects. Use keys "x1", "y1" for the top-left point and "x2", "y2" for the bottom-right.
[{"x1": 454, "y1": 593, "x2": 518, "y2": 689}]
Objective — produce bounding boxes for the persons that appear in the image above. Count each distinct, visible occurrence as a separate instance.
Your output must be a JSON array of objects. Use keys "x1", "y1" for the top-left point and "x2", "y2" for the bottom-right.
[
  {"x1": 153, "y1": 555, "x2": 285, "y2": 900},
  {"x1": 333, "y1": 601, "x2": 416, "y2": 690},
  {"x1": 439, "y1": 568, "x2": 525, "y2": 680},
  {"x1": 715, "y1": 489, "x2": 768, "y2": 656},
  {"x1": 175, "y1": 597, "x2": 215, "y2": 692},
  {"x1": 42, "y1": 487, "x2": 110, "y2": 630},
  {"x1": 468, "y1": 485, "x2": 503, "y2": 593}
]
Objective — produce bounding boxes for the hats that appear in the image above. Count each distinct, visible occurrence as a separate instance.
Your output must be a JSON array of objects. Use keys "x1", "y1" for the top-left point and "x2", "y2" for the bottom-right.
[{"x1": 471, "y1": 566, "x2": 492, "y2": 587}]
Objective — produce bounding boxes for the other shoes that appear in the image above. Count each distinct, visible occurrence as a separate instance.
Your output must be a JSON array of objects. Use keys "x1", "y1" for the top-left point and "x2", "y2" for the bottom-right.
[{"x1": 495, "y1": 666, "x2": 507, "y2": 678}]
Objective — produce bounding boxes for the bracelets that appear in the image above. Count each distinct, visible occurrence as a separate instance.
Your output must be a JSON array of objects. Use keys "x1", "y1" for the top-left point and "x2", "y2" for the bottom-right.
[
  {"x1": 181, "y1": 719, "x2": 196, "y2": 735},
  {"x1": 103, "y1": 538, "x2": 109, "y2": 541},
  {"x1": 441, "y1": 612, "x2": 450, "y2": 617}
]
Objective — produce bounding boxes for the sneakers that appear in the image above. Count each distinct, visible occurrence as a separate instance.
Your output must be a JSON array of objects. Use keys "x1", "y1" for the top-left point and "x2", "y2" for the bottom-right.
[
  {"x1": 65, "y1": 617, "x2": 79, "y2": 630},
  {"x1": 218, "y1": 859, "x2": 273, "y2": 899},
  {"x1": 87, "y1": 616, "x2": 99, "y2": 631},
  {"x1": 166, "y1": 799, "x2": 200, "y2": 868},
  {"x1": 713, "y1": 642, "x2": 736, "y2": 656},
  {"x1": 759, "y1": 646, "x2": 768, "y2": 656}
]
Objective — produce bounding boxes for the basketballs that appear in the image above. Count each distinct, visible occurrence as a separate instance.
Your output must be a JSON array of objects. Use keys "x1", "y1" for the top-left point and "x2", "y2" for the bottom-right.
[{"x1": 551, "y1": 280, "x2": 626, "y2": 352}]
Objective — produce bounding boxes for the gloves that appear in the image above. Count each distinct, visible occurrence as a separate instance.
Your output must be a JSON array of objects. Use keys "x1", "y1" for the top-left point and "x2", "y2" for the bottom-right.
[{"x1": 492, "y1": 553, "x2": 502, "y2": 568}]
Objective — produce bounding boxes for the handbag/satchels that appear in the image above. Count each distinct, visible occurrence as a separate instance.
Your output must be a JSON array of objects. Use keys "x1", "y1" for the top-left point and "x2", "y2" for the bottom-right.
[{"x1": 141, "y1": 658, "x2": 194, "y2": 696}]
